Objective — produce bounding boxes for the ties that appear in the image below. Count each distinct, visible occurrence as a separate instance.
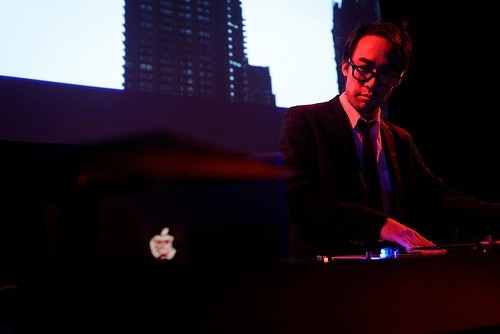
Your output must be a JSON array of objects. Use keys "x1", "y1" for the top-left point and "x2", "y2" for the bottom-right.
[{"x1": 357, "y1": 121, "x2": 383, "y2": 210}]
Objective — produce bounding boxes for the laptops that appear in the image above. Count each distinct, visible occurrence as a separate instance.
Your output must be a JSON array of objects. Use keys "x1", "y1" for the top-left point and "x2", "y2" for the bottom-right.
[{"x1": 48, "y1": 150, "x2": 294, "y2": 269}]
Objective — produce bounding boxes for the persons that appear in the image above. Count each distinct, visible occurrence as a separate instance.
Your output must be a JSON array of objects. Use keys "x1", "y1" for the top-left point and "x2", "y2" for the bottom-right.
[{"x1": 276, "y1": 18, "x2": 499, "y2": 257}]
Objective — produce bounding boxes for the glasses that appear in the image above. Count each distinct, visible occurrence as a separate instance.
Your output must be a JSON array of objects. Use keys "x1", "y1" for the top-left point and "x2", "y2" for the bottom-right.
[{"x1": 346, "y1": 54, "x2": 402, "y2": 87}]
